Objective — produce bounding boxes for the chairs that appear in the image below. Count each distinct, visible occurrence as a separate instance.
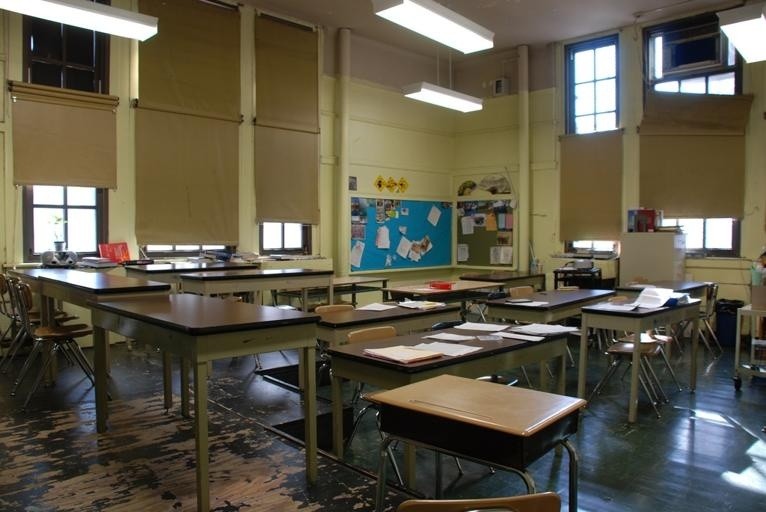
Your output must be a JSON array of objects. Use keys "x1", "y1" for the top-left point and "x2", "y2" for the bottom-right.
[
  {"x1": 396, "y1": 491, "x2": 562, "y2": 512},
  {"x1": 156, "y1": 273, "x2": 618, "y2": 395},
  {"x1": 341, "y1": 325, "x2": 405, "y2": 487},
  {"x1": 428, "y1": 320, "x2": 465, "y2": 329},
  {"x1": 1, "y1": 272, "x2": 113, "y2": 411},
  {"x1": 587, "y1": 297, "x2": 691, "y2": 419}
]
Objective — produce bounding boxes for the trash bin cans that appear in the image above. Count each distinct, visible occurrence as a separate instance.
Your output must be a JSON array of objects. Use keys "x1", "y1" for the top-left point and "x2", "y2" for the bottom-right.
[{"x1": 712, "y1": 298, "x2": 743, "y2": 345}]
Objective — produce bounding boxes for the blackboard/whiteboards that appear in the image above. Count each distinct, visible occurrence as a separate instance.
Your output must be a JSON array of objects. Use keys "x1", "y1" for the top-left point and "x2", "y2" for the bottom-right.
[
  {"x1": 456, "y1": 200, "x2": 513, "y2": 269},
  {"x1": 350, "y1": 199, "x2": 456, "y2": 272}
]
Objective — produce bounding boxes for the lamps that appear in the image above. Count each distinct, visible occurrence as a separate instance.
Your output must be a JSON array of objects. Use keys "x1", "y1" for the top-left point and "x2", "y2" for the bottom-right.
[
  {"x1": 403, "y1": 43, "x2": 484, "y2": 114},
  {"x1": 715, "y1": 0, "x2": 766, "y2": 65},
  {"x1": 374, "y1": 2, "x2": 494, "y2": 56},
  {"x1": 0, "y1": 1, "x2": 160, "y2": 42}
]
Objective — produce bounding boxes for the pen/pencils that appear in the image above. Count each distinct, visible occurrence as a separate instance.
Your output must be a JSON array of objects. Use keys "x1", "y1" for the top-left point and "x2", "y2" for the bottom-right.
[{"x1": 117, "y1": 260, "x2": 154, "y2": 265}]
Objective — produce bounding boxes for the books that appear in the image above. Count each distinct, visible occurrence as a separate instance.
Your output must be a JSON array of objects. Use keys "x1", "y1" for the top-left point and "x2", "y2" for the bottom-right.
[
  {"x1": 664, "y1": 292, "x2": 691, "y2": 307},
  {"x1": 364, "y1": 345, "x2": 443, "y2": 362},
  {"x1": 81, "y1": 249, "x2": 322, "y2": 269}
]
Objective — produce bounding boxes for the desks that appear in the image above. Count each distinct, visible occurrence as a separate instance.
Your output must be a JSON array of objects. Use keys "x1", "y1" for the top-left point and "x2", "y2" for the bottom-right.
[
  {"x1": 13, "y1": 269, "x2": 174, "y2": 411},
  {"x1": 618, "y1": 276, "x2": 719, "y2": 364},
  {"x1": 86, "y1": 293, "x2": 322, "y2": 511},
  {"x1": 327, "y1": 320, "x2": 570, "y2": 486},
  {"x1": 578, "y1": 296, "x2": 702, "y2": 422},
  {"x1": 360, "y1": 372, "x2": 588, "y2": 511}
]
